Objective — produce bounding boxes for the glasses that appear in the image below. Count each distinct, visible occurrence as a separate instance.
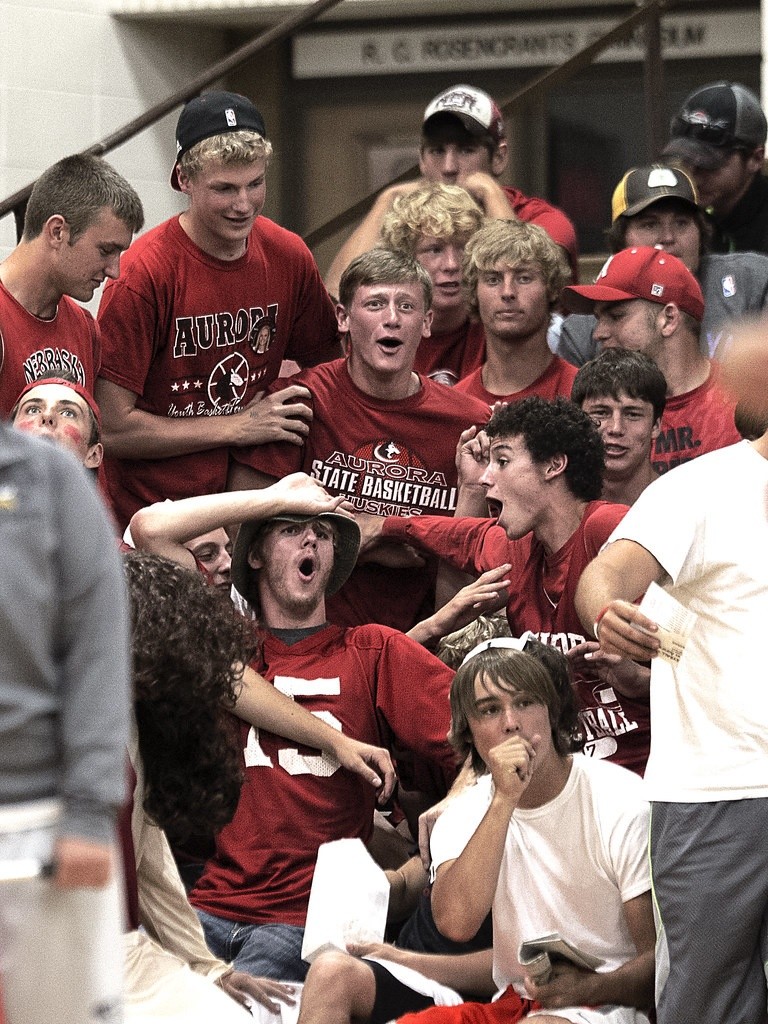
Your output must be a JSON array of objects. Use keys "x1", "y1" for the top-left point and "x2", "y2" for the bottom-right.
[{"x1": 670, "y1": 117, "x2": 736, "y2": 147}]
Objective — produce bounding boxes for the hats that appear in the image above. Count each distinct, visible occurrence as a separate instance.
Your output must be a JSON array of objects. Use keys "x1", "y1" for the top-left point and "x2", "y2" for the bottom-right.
[
  {"x1": 421, "y1": 85, "x2": 505, "y2": 143},
  {"x1": 559, "y1": 246, "x2": 706, "y2": 321},
  {"x1": 611, "y1": 166, "x2": 698, "y2": 226},
  {"x1": 12, "y1": 373, "x2": 101, "y2": 476},
  {"x1": 171, "y1": 91, "x2": 266, "y2": 190},
  {"x1": 661, "y1": 81, "x2": 768, "y2": 168},
  {"x1": 230, "y1": 512, "x2": 361, "y2": 598}
]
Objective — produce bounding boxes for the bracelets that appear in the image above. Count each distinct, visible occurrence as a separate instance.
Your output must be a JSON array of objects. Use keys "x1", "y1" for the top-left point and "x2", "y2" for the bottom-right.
[{"x1": 594, "y1": 607, "x2": 610, "y2": 639}]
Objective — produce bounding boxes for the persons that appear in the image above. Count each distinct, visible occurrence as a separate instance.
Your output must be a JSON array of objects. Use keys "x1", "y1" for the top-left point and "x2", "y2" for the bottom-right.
[
  {"x1": 447, "y1": 219, "x2": 578, "y2": 406},
  {"x1": 573, "y1": 349, "x2": 664, "y2": 508},
  {"x1": 559, "y1": 246, "x2": 747, "y2": 476},
  {"x1": 230, "y1": 248, "x2": 507, "y2": 649},
  {"x1": 131, "y1": 470, "x2": 458, "y2": 993},
  {"x1": 338, "y1": 180, "x2": 488, "y2": 388},
  {"x1": 662, "y1": 82, "x2": 768, "y2": 256},
  {"x1": 119, "y1": 501, "x2": 655, "y2": 1024},
  {"x1": 575, "y1": 420, "x2": 768, "y2": 1024},
  {"x1": 0, "y1": 154, "x2": 144, "y2": 501},
  {"x1": 10, "y1": 370, "x2": 104, "y2": 480},
  {"x1": 94, "y1": 92, "x2": 345, "y2": 535},
  {"x1": 0, "y1": 424, "x2": 132, "y2": 1024},
  {"x1": 345, "y1": 399, "x2": 651, "y2": 782},
  {"x1": 548, "y1": 165, "x2": 768, "y2": 359},
  {"x1": 321, "y1": 84, "x2": 579, "y2": 318}
]
